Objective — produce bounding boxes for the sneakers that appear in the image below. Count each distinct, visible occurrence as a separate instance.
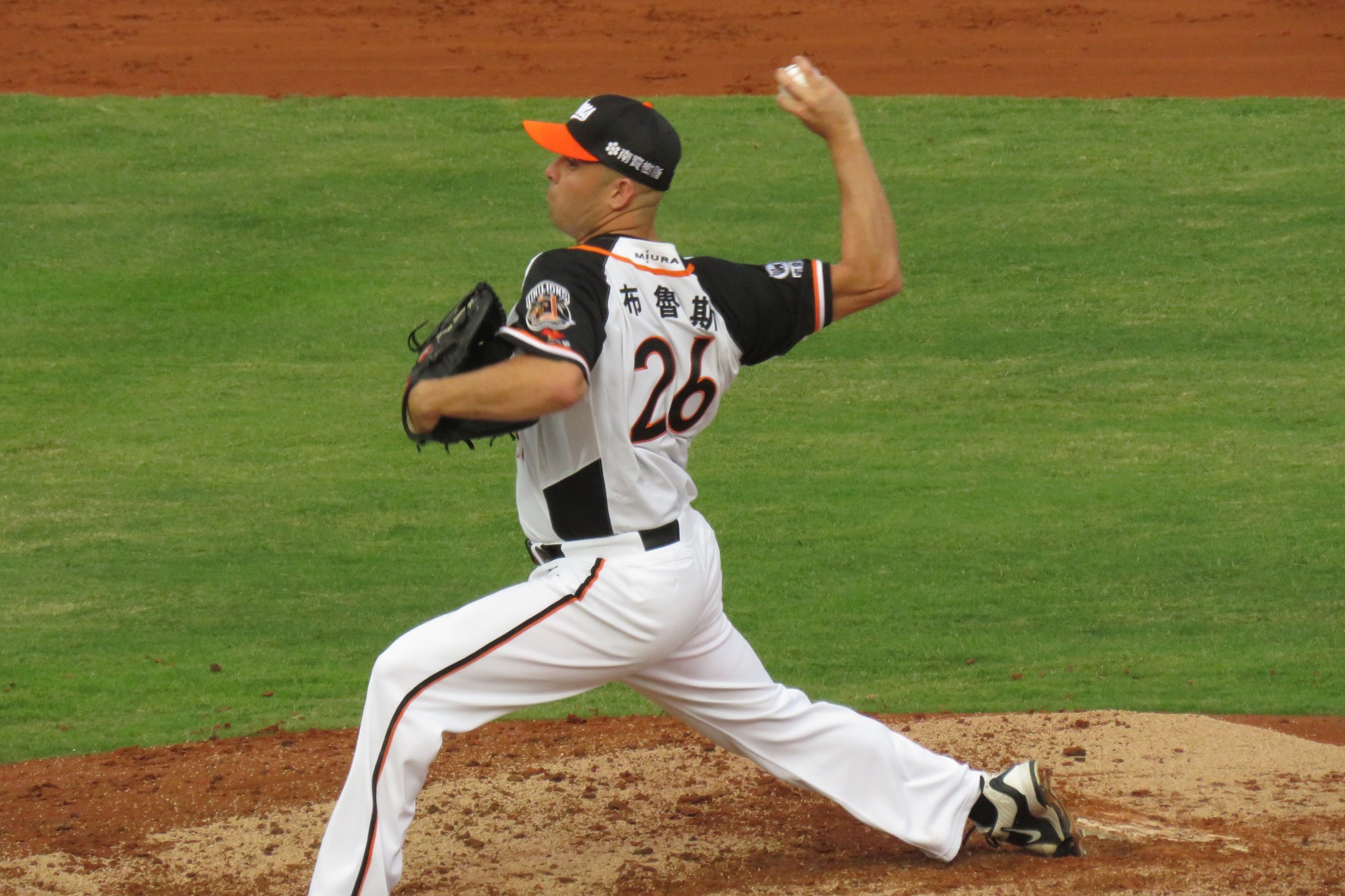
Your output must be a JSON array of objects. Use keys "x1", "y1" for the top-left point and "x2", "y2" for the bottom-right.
[{"x1": 962, "y1": 759, "x2": 1084, "y2": 859}]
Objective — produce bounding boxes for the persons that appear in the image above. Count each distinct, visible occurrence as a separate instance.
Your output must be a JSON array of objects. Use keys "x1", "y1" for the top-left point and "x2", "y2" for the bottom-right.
[{"x1": 307, "y1": 55, "x2": 1105, "y2": 896}]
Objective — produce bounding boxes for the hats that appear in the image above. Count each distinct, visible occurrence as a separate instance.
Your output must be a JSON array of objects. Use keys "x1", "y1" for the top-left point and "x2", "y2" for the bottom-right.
[{"x1": 521, "y1": 94, "x2": 683, "y2": 191}]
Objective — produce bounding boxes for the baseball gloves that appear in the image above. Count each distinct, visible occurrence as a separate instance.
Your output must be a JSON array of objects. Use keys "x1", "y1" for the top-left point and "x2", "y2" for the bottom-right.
[{"x1": 400, "y1": 280, "x2": 542, "y2": 452}]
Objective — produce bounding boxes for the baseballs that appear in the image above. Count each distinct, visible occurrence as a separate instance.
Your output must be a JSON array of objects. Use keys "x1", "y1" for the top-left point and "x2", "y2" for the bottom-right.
[{"x1": 778, "y1": 63, "x2": 822, "y2": 96}]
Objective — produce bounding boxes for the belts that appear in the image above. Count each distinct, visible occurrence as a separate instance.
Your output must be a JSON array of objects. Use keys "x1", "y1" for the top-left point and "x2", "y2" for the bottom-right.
[{"x1": 538, "y1": 519, "x2": 680, "y2": 564}]
